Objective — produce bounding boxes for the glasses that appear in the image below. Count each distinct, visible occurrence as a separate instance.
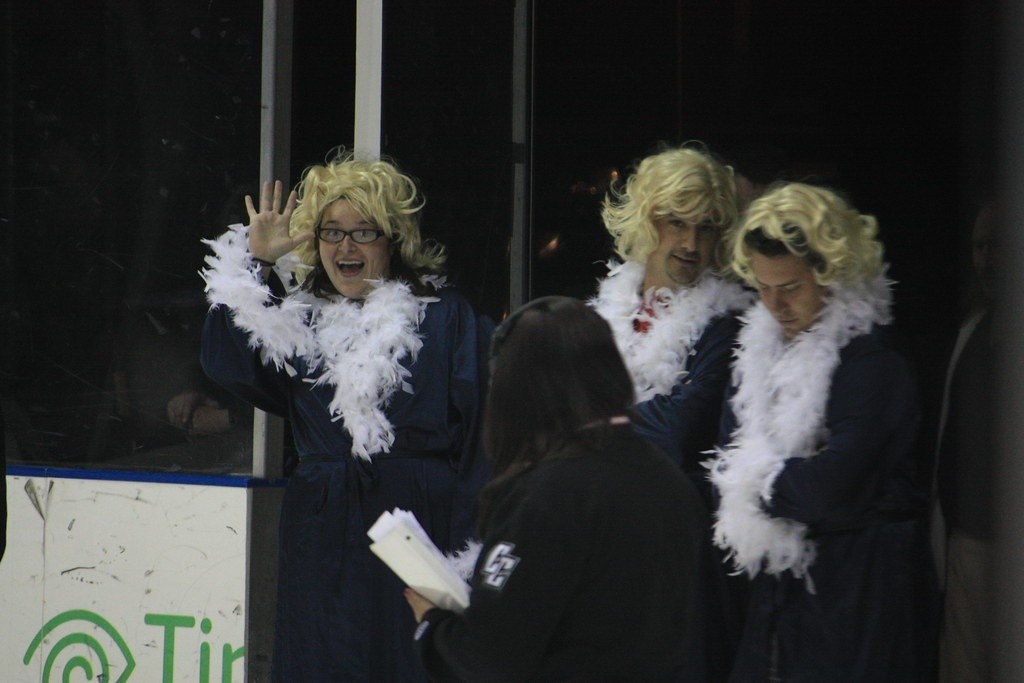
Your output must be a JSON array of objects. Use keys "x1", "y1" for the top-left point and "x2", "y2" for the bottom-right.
[{"x1": 315, "y1": 226, "x2": 385, "y2": 244}]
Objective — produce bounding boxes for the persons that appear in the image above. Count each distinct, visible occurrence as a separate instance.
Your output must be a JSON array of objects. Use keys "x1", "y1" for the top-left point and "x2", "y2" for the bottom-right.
[
  {"x1": 405, "y1": 296, "x2": 707, "y2": 682},
  {"x1": 707, "y1": 182, "x2": 938, "y2": 682},
  {"x1": 938, "y1": 203, "x2": 1004, "y2": 683},
  {"x1": 114, "y1": 308, "x2": 237, "y2": 446},
  {"x1": 589, "y1": 149, "x2": 748, "y2": 488},
  {"x1": 198, "y1": 159, "x2": 497, "y2": 683}
]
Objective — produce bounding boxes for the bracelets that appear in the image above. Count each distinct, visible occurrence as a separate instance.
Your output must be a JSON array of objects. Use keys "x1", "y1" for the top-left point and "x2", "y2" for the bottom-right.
[{"x1": 251, "y1": 257, "x2": 276, "y2": 267}]
